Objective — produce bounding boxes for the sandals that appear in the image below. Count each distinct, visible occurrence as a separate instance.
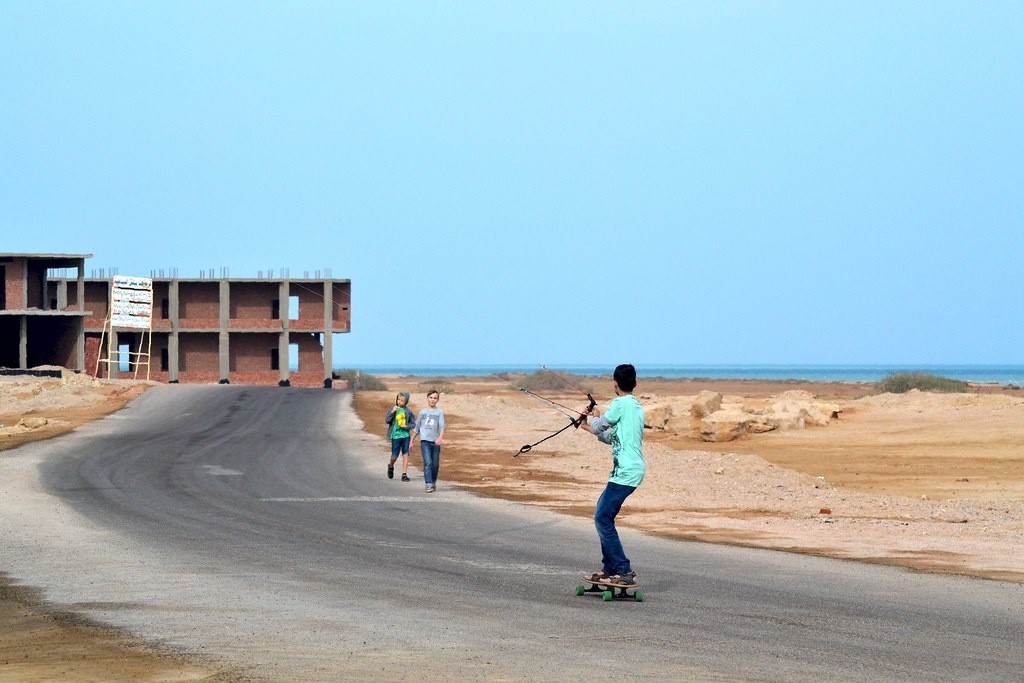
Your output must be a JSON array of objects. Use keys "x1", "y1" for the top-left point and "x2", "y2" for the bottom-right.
[{"x1": 583, "y1": 570, "x2": 636, "y2": 585}]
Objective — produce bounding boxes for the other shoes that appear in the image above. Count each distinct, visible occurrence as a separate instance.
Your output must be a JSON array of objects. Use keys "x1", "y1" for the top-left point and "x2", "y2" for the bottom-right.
[
  {"x1": 388, "y1": 464, "x2": 394, "y2": 479},
  {"x1": 426, "y1": 487, "x2": 436, "y2": 492},
  {"x1": 401, "y1": 476, "x2": 410, "y2": 482}
]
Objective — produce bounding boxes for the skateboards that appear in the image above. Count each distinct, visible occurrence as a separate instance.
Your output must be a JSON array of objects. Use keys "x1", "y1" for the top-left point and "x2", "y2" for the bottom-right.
[{"x1": 575, "y1": 574, "x2": 645, "y2": 603}]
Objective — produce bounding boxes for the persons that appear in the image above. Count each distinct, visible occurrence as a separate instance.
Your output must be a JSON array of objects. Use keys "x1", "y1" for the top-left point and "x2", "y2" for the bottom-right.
[
  {"x1": 409, "y1": 389, "x2": 446, "y2": 493},
  {"x1": 578, "y1": 363, "x2": 646, "y2": 586},
  {"x1": 385, "y1": 391, "x2": 416, "y2": 482}
]
